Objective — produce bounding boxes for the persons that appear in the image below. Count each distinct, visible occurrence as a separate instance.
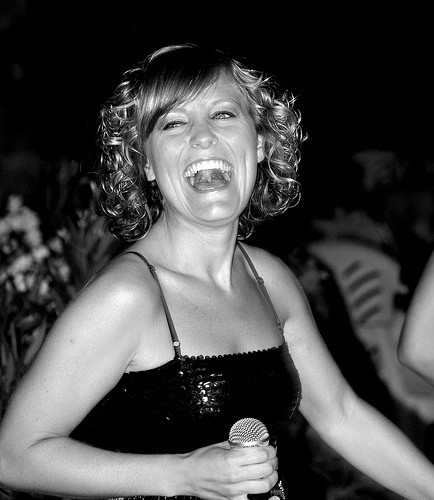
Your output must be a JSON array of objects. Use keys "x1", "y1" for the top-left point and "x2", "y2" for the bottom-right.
[
  {"x1": 394, "y1": 249, "x2": 434, "y2": 387},
  {"x1": 0, "y1": 40, "x2": 434, "y2": 499},
  {"x1": 304, "y1": 143, "x2": 433, "y2": 315}
]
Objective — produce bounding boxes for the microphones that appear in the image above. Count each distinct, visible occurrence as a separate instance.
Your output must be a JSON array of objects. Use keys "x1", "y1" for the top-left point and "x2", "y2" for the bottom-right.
[{"x1": 227, "y1": 417, "x2": 279, "y2": 500}]
